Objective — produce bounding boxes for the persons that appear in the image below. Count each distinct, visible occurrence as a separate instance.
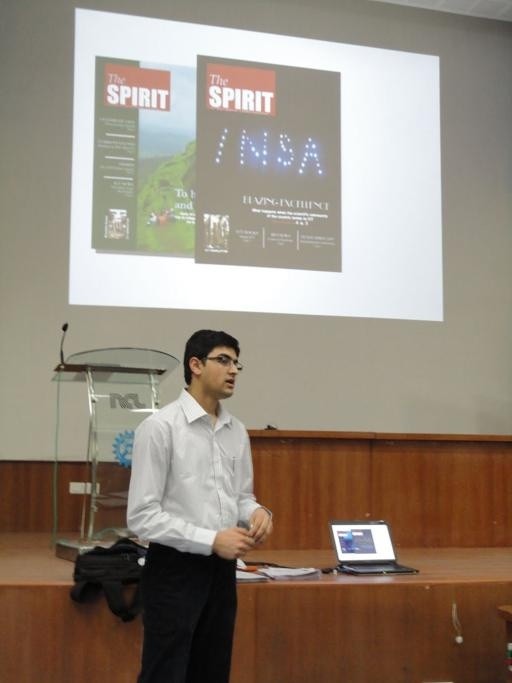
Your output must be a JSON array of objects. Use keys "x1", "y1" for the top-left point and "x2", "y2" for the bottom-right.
[{"x1": 126, "y1": 329, "x2": 275, "y2": 682}]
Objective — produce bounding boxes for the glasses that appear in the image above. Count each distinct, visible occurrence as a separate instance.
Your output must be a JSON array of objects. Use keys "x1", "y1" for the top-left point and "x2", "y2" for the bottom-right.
[{"x1": 200, "y1": 353, "x2": 243, "y2": 372}]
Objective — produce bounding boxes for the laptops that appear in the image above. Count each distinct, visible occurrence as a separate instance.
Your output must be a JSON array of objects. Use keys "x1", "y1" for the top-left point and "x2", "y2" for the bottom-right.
[{"x1": 329, "y1": 521, "x2": 421, "y2": 577}]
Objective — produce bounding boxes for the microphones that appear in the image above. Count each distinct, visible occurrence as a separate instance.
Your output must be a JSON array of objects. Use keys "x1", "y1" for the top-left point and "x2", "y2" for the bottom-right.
[{"x1": 57, "y1": 323, "x2": 68, "y2": 365}]
